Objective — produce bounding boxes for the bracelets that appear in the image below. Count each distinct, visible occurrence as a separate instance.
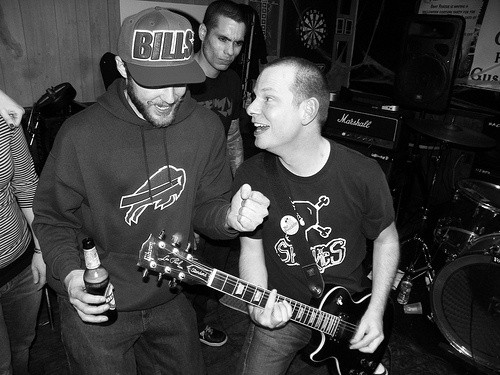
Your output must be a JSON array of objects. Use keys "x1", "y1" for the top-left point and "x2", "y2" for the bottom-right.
[{"x1": 34, "y1": 248, "x2": 41, "y2": 254}]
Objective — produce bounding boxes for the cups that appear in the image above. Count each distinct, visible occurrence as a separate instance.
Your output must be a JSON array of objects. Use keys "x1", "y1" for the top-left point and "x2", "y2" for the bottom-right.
[{"x1": 404, "y1": 302, "x2": 423, "y2": 314}]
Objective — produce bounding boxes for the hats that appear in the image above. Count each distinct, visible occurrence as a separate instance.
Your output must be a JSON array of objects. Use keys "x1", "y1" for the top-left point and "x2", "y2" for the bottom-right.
[{"x1": 117, "y1": 5, "x2": 206, "y2": 86}]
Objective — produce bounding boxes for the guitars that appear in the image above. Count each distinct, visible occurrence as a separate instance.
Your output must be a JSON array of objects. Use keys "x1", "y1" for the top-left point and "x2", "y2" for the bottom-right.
[{"x1": 134, "y1": 227, "x2": 403, "y2": 375}]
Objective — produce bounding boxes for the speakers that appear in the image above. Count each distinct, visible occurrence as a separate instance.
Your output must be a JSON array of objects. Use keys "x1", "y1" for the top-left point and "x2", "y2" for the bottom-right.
[
  {"x1": 320, "y1": 131, "x2": 403, "y2": 221},
  {"x1": 331, "y1": 0, "x2": 467, "y2": 114}
]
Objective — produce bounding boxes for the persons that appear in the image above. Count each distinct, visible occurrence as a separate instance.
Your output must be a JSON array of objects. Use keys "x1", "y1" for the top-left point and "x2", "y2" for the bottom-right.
[
  {"x1": 230, "y1": 57, "x2": 400, "y2": 375},
  {"x1": 188, "y1": 0, "x2": 249, "y2": 346},
  {"x1": 0, "y1": 89, "x2": 47, "y2": 375},
  {"x1": 32, "y1": 6, "x2": 270, "y2": 375}
]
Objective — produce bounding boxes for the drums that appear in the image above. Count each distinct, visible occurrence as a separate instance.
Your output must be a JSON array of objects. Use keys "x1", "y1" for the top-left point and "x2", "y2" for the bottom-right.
[
  {"x1": 446, "y1": 178, "x2": 500, "y2": 236},
  {"x1": 430, "y1": 231, "x2": 500, "y2": 369},
  {"x1": 433, "y1": 225, "x2": 482, "y2": 265}
]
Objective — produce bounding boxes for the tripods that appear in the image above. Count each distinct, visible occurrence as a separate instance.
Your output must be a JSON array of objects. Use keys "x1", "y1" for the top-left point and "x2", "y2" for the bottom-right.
[{"x1": 390, "y1": 141, "x2": 447, "y2": 305}]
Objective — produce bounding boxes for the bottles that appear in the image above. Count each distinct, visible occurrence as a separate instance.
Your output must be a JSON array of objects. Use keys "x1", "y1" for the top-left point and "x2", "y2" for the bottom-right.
[
  {"x1": 397, "y1": 274, "x2": 412, "y2": 305},
  {"x1": 82, "y1": 237, "x2": 118, "y2": 327}
]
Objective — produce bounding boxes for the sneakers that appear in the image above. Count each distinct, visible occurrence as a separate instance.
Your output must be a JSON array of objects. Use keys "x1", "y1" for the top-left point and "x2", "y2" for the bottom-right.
[
  {"x1": 199, "y1": 325, "x2": 228, "y2": 346},
  {"x1": 360, "y1": 359, "x2": 388, "y2": 375}
]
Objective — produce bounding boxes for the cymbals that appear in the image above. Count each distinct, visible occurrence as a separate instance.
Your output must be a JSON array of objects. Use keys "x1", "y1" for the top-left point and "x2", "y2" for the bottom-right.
[{"x1": 405, "y1": 119, "x2": 497, "y2": 148}]
